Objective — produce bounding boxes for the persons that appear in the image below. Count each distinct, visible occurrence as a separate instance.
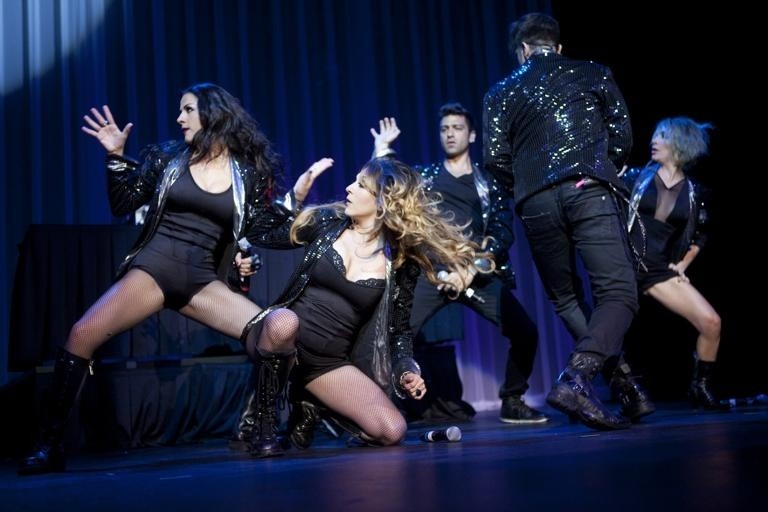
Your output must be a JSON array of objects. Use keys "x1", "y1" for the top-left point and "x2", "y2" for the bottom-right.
[
  {"x1": 14, "y1": 83, "x2": 336, "y2": 477},
  {"x1": 481, "y1": 14, "x2": 656, "y2": 430},
  {"x1": 369, "y1": 100, "x2": 551, "y2": 426},
  {"x1": 619, "y1": 116, "x2": 732, "y2": 412},
  {"x1": 241, "y1": 154, "x2": 499, "y2": 458}
]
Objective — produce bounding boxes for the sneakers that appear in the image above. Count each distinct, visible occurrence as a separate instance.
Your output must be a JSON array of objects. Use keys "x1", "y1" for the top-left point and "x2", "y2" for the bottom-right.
[{"x1": 499, "y1": 394, "x2": 550, "y2": 422}]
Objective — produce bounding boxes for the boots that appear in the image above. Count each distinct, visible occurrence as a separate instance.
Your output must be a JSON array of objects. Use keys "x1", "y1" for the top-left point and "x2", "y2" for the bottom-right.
[
  {"x1": 25, "y1": 348, "x2": 94, "y2": 471},
  {"x1": 547, "y1": 352, "x2": 633, "y2": 429},
  {"x1": 231, "y1": 344, "x2": 382, "y2": 458},
  {"x1": 689, "y1": 353, "x2": 734, "y2": 411},
  {"x1": 609, "y1": 362, "x2": 655, "y2": 418}
]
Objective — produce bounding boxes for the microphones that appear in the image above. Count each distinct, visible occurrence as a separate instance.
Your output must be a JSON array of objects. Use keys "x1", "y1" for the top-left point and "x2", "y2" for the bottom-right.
[
  {"x1": 437, "y1": 270, "x2": 486, "y2": 306},
  {"x1": 237, "y1": 236, "x2": 256, "y2": 296},
  {"x1": 719, "y1": 393, "x2": 767, "y2": 412},
  {"x1": 419, "y1": 425, "x2": 463, "y2": 444}
]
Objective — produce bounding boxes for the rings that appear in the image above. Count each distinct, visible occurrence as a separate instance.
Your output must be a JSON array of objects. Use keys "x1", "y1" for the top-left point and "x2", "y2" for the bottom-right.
[
  {"x1": 102, "y1": 120, "x2": 109, "y2": 126},
  {"x1": 416, "y1": 388, "x2": 422, "y2": 397}
]
